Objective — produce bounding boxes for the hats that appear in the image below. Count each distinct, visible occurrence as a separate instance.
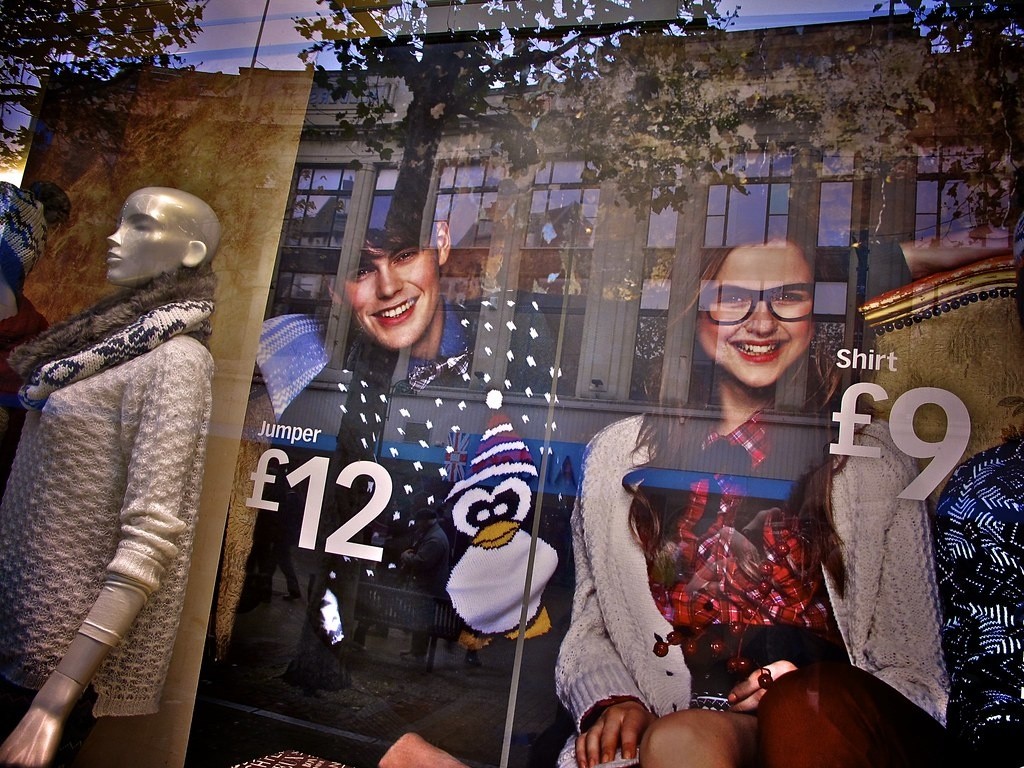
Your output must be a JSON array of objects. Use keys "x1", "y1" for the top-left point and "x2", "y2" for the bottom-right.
[{"x1": 0, "y1": 180, "x2": 72, "y2": 295}]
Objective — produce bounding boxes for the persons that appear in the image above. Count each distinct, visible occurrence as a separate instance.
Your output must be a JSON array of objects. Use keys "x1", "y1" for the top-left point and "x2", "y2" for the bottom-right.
[
  {"x1": 930, "y1": 206, "x2": 1024, "y2": 768},
  {"x1": 553, "y1": 208, "x2": 959, "y2": 768},
  {"x1": 213, "y1": 169, "x2": 1011, "y2": 768},
  {"x1": 0, "y1": 178, "x2": 75, "y2": 489},
  {"x1": 0, "y1": 187, "x2": 222, "y2": 768}
]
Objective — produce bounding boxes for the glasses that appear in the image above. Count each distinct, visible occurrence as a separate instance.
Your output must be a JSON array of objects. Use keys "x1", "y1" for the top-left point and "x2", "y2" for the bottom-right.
[{"x1": 702, "y1": 282, "x2": 815, "y2": 324}]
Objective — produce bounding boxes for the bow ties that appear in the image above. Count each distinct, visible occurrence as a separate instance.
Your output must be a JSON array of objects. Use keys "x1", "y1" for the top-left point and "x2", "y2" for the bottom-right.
[{"x1": 406, "y1": 353, "x2": 473, "y2": 394}]
